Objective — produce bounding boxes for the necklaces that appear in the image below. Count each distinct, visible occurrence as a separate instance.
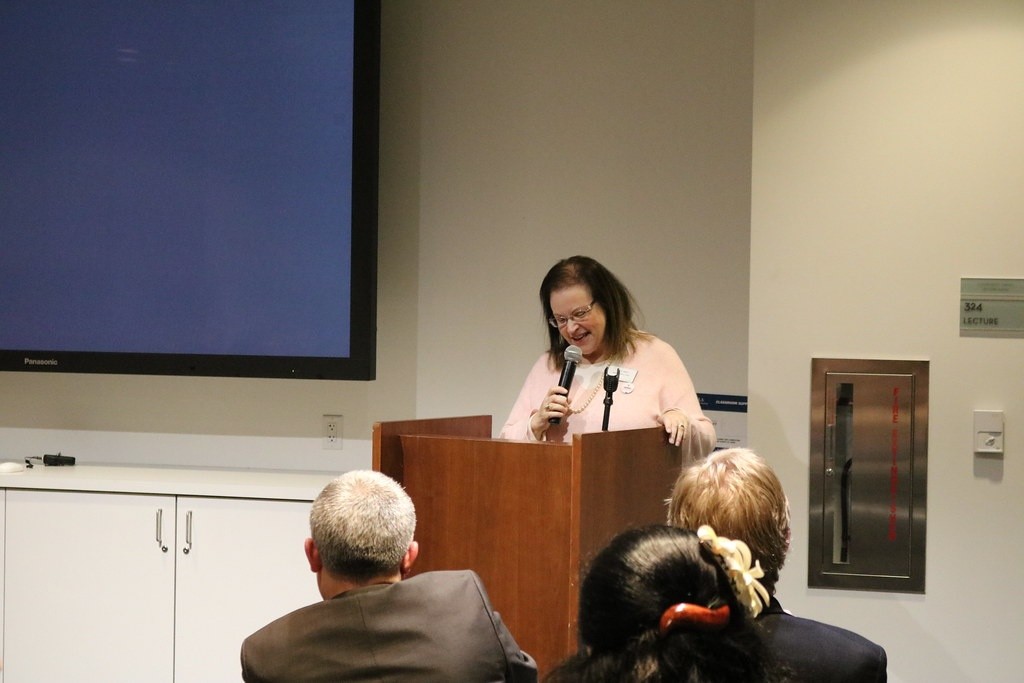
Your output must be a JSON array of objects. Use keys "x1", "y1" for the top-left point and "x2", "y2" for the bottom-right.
[{"x1": 556, "y1": 344, "x2": 619, "y2": 415}]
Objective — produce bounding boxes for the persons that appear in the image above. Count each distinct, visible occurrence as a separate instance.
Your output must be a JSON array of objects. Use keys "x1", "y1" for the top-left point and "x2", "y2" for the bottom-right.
[
  {"x1": 657, "y1": 444, "x2": 889, "y2": 683},
  {"x1": 237, "y1": 467, "x2": 541, "y2": 683},
  {"x1": 538, "y1": 521, "x2": 809, "y2": 683},
  {"x1": 495, "y1": 253, "x2": 719, "y2": 468}
]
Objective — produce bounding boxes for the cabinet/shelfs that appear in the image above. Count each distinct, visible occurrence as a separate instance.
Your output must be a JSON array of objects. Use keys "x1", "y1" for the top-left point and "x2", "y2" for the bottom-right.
[{"x1": 0, "y1": 487, "x2": 323, "y2": 683}]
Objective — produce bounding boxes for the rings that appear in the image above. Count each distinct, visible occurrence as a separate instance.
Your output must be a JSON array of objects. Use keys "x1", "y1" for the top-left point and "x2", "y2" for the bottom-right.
[
  {"x1": 679, "y1": 423, "x2": 686, "y2": 428},
  {"x1": 544, "y1": 403, "x2": 553, "y2": 411}
]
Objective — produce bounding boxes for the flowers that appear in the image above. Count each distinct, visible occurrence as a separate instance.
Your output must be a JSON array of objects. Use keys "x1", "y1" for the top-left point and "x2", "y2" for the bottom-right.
[{"x1": 696, "y1": 525, "x2": 770, "y2": 619}]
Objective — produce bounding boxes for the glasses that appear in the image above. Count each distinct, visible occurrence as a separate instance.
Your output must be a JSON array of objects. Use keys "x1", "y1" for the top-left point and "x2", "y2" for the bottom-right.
[{"x1": 546, "y1": 299, "x2": 597, "y2": 328}]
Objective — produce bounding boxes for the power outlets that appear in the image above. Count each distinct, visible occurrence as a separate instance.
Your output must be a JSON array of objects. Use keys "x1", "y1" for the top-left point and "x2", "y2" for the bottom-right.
[{"x1": 323, "y1": 415, "x2": 343, "y2": 450}]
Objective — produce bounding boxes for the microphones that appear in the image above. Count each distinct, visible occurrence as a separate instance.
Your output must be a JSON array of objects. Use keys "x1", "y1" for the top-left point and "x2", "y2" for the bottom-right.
[{"x1": 549, "y1": 345, "x2": 583, "y2": 424}]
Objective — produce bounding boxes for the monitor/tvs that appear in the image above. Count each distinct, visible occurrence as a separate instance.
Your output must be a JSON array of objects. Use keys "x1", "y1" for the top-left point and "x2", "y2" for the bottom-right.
[{"x1": 0, "y1": 0, "x2": 382, "y2": 381}]
